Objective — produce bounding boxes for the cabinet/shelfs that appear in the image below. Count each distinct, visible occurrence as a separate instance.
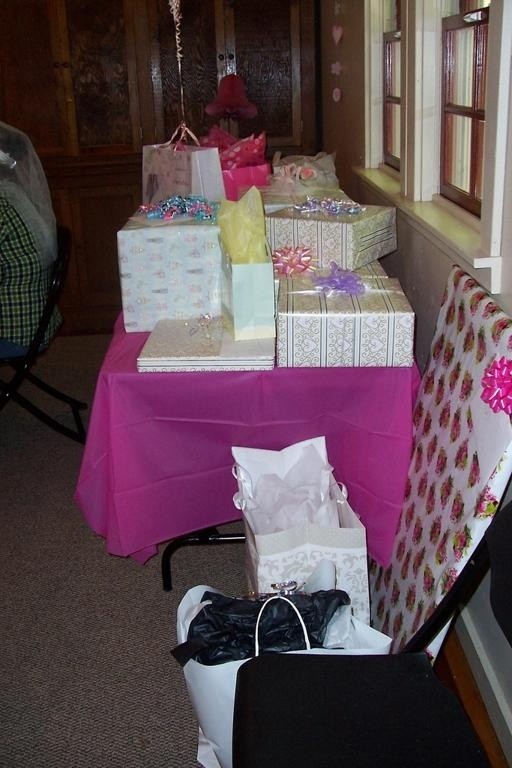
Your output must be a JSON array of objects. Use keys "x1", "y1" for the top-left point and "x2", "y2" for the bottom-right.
[{"x1": 3, "y1": 0, "x2": 319, "y2": 337}]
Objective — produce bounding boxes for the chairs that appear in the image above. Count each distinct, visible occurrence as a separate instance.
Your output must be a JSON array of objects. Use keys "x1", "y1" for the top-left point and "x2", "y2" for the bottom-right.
[
  {"x1": 233, "y1": 504, "x2": 512, "y2": 766},
  {"x1": 0, "y1": 220, "x2": 88, "y2": 445}
]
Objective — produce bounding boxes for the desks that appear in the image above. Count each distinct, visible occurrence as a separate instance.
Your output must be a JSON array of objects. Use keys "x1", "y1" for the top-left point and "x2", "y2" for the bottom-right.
[{"x1": 99, "y1": 277, "x2": 426, "y2": 591}]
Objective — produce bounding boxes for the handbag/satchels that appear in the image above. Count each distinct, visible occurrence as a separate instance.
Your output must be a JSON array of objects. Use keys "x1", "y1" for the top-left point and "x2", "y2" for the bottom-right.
[
  {"x1": 174, "y1": 584, "x2": 394, "y2": 768},
  {"x1": 218, "y1": 235, "x2": 276, "y2": 343},
  {"x1": 142, "y1": 123, "x2": 225, "y2": 215},
  {"x1": 229, "y1": 436, "x2": 372, "y2": 627},
  {"x1": 231, "y1": 650, "x2": 493, "y2": 767}
]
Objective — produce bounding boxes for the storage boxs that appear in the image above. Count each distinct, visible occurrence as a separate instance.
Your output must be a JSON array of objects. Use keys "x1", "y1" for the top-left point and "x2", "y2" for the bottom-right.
[
  {"x1": 275, "y1": 276, "x2": 416, "y2": 365},
  {"x1": 254, "y1": 184, "x2": 353, "y2": 232},
  {"x1": 116, "y1": 206, "x2": 226, "y2": 334},
  {"x1": 274, "y1": 263, "x2": 391, "y2": 281},
  {"x1": 264, "y1": 199, "x2": 399, "y2": 271}
]
